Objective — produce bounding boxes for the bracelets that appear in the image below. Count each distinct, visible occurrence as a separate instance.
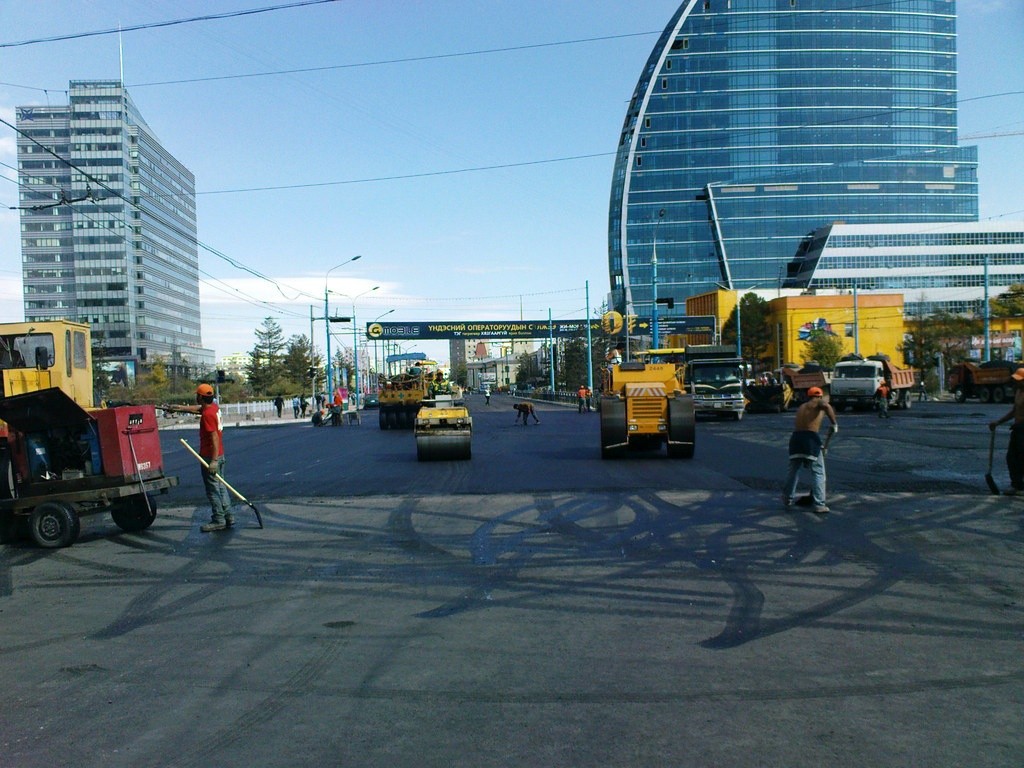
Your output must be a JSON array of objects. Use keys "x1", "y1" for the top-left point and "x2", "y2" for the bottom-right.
[{"x1": 212, "y1": 458, "x2": 218, "y2": 461}]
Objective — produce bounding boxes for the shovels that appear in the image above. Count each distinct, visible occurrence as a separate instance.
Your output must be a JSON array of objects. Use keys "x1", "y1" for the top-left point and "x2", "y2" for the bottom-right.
[
  {"x1": 985, "y1": 428, "x2": 999, "y2": 495},
  {"x1": 795, "y1": 428, "x2": 833, "y2": 506},
  {"x1": 180, "y1": 438, "x2": 264, "y2": 529}
]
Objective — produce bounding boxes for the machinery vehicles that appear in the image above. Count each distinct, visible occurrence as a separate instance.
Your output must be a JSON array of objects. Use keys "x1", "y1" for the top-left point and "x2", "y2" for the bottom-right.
[
  {"x1": 0, "y1": 317, "x2": 103, "y2": 434},
  {"x1": 362, "y1": 351, "x2": 473, "y2": 461},
  {"x1": 597, "y1": 344, "x2": 746, "y2": 461}
]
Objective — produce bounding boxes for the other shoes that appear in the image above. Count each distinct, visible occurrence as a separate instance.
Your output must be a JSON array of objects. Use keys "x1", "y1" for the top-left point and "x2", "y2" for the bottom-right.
[
  {"x1": 226, "y1": 522, "x2": 237, "y2": 529},
  {"x1": 199, "y1": 522, "x2": 226, "y2": 532},
  {"x1": 1003, "y1": 486, "x2": 1024, "y2": 496},
  {"x1": 782, "y1": 494, "x2": 793, "y2": 506},
  {"x1": 878, "y1": 416, "x2": 883, "y2": 418},
  {"x1": 813, "y1": 507, "x2": 830, "y2": 513},
  {"x1": 885, "y1": 415, "x2": 891, "y2": 419}
]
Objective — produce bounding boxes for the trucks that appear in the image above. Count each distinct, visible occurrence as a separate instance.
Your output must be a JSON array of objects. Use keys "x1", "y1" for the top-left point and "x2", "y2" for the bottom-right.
[
  {"x1": 947, "y1": 356, "x2": 1024, "y2": 403},
  {"x1": 830, "y1": 355, "x2": 915, "y2": 413}
]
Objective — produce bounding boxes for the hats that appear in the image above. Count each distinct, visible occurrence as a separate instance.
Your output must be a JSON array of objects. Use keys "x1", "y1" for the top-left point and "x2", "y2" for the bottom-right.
[
  {"x1": 1011, "y1": 367, "x2": 1024, "y2": 381},
  {"x1": 437, "y1": 370, "x2": 443, "y2": 375},
  {"x1": 321, "y1": 409, "x2": 325, "y2": 414},
  {"x1": 880, "y1": 380, "x2": 886, "y2": 385},
  {"x1": 191, "y1": 384, "x2": 214, "y2": 396},
  {"x1": 579, "y1": 385, "x2": 584, "y2": 388},
  {"x1": 807, "y1": 386, "x2": 823, "y2": 397},
  {"x1": 486, "y1": 384, "x2": 491, "y2": 388}
]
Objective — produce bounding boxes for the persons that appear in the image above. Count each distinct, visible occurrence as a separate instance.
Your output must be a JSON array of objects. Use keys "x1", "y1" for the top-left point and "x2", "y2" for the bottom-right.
[
  {"x1": 989, "y1": 368, "x2": 1024, "y2": 496},
  {"x1": 311, "y1": 410, "x2": 325, "y2": 427},
  {"x1": 578, "y1": 386, "x2": 593, "y2": 413},
  {"x1": 485, "y1": 387, "x2": 491, "y2": 405},
  {"x1": 873, "y1": 382, "x2": 890, "y2": 419},
  {"x1": 760, "y1": 373, "x2": 778, "y2": 385},
  {"x1": 316, "y1": 393, "x2": 325, "y2": 411},
  {"x1": 275, "y1": 392, "x2": 284, "y2": 419},
  {"x1": 409, "y1": 362, "x2": 422, "y2": 375},
  {"x1": 428, "y1": 373, "x2": 452, "y2": 398},
  {"x1": 293, "y1": 395, "x2": 300, "y2": 419},
  {"x1": 513, "y1": 402, "x2": 541, "y2": 425},
  {"x1": 782, "y1": 386, "x2": 838, "y2": 512},
  {"x1": 326, "y1": 392, "x2": 344, "y2": 425},
  {"x1": 606, "y1": 342, "x2": 625, "y2": 373},
  {"x1": 193, "y1": 383, "x2": 236, "y2": 533},
  {"x1": 493, "y1": 386, "x2": 516, "y2": 398},
  {"x1": 919, "y1": 381, "x2": 927, "y2": 400},
  {"x1": 301, "y1": 395, "x2": 309, "y2": 418},
  {"x1": 539, "y1": 385, "x2": 566, "y2": 401}
]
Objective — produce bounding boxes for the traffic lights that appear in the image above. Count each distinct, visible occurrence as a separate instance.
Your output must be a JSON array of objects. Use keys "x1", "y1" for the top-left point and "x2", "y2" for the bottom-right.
[{"x1": 311, "y1": 366, "x2": 318, "y2": 378}]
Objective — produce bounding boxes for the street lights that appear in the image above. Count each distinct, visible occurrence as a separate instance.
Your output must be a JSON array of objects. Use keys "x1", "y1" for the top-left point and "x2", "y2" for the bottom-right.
[
  {"x1": 652, "y1": 208, "x2": 668, "y2": 364},
  {"x1": 714, "y1": 282, "x2": 765, "y2": 357},
  {"x1": 374, "y1": 309, "x2": 395, "y2": 393},
  {"x1": 325, "y1": 255, "x2": 361, "y2": 413},
  {"x1": 328, "y1": 286, "x2": 379, "y2": 410}
]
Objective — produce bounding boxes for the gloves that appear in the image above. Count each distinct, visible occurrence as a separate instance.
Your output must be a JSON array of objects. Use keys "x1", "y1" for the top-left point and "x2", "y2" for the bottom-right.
[
  {"x1": 208, "y1": 458, "x2": 220, "y2": 476},
  {"x1": 165, "y1": 404, "x2": 181, "y2": 414}
]
